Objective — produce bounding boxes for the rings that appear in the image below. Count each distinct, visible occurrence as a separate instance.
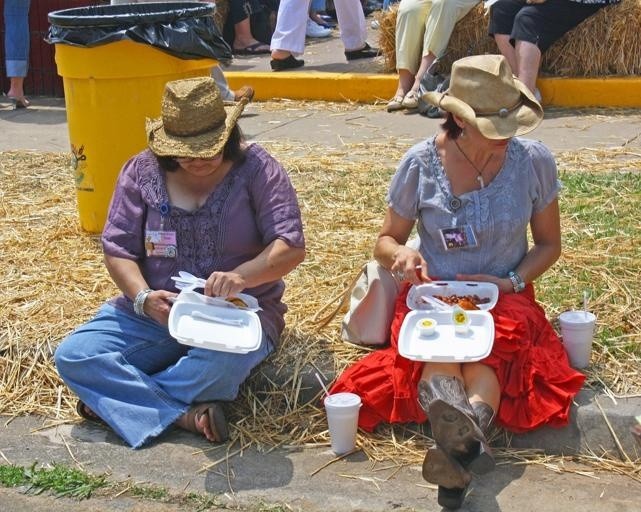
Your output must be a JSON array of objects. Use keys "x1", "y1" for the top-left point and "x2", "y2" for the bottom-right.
[{"x1": 398, "y1": 272, "x2": 405, "y2": 281}]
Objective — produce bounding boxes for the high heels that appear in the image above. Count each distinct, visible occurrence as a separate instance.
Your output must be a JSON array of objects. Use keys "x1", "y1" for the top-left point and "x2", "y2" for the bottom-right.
[{"x1": 8, "y1": 96, "x2": 30, "y2": 111}]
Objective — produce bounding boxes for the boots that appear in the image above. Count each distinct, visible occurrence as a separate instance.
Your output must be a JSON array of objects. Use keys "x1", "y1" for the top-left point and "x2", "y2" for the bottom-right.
[{"x1": 416, "y1": 374, "x2": 495, "y2": 510}]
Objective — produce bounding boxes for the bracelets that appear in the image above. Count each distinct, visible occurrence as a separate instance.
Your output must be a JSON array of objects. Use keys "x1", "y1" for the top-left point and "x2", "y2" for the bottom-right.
[
  {"x1": 510, "y1": 276, "x2": 521, "y2": 294},
  {"x1": 508, "y1": 271, "x2": 526, "y2": 290},
  {"x1": 133, "y1": 289, "x2": 154, "y2": 315},
  {"x1": 138, "y1": 292, "x2": 151, "y2": 318}
]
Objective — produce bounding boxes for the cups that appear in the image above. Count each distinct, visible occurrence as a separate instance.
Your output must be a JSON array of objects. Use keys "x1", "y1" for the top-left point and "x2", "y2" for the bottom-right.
[
  {"x1": 558, "y1": 310, "x2": 596, "y2": 371},
  {"x1": 323, "y1": 392, "x2": 361, "y2": 454}
]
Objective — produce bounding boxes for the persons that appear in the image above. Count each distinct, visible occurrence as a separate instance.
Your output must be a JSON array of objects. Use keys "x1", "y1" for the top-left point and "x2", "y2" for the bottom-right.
[
  {"x1": 229, "y1": 1, "x2": 397, "y2": 57},
  {"x1": 387, "y1": 0, "x2": 485, "y2": 110},
  {"x1": 320, "y1": 52, "x2": 587, "y2": 512},
  {"x1": 488, "y1": 0, "x2": 624, "y2": 104},
  {"x1": 0, "y1": 1, "x2": 33, "y2": 111},
  {"x1": 269, "y1": 0, "x2": 382, "y2": 69},
  {"x1": 52, "y1": 75, "x2": 308, "y2": 452}
]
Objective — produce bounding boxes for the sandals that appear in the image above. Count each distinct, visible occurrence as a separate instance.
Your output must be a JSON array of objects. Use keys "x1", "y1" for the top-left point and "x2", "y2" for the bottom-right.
[
  {"x1": 386, "y1": 89, "x2": 424, "y2": 113},
  {"x1": 178, "y1": 400, "x2": 231, "y2": 447},
  {"x1": 74, "y1": 401, "x2": 106, "y2": 423}
]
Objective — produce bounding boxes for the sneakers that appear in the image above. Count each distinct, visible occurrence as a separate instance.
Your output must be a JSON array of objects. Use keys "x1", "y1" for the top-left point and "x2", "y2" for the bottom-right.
[
  {"x1": 233, "y1": 86, "x2": 256, "y2": 106},
  {"x1": 302, "y1": 18, "x2": 334, "y2": 39}
]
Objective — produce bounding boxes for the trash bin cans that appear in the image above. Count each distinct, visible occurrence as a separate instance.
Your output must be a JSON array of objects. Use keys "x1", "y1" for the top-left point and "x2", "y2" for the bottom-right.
[{"x1": 47, "y1": 2, "x2": 223, "y2": 233}]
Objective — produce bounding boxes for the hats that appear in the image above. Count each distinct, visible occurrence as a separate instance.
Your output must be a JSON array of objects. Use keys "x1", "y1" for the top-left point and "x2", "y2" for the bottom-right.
[
  {"x1": 422, "y1": 53, "x2": 545, "y2": 141},
  {"x1": 144, "y1": 76, "x2": 247, "y2": 161}
]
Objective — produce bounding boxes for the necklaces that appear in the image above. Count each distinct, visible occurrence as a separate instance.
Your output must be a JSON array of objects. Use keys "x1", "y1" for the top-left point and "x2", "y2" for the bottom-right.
[{"x1": 453, "y1": 138, "x2": 494, "y2": 190}]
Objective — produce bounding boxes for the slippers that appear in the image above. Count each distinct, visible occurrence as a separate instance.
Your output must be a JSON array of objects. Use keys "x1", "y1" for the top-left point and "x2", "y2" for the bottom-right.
[
  {"x1": 231, "y1": 39, "x2": 274, "y2": 55},
  {"x1": 270, "y1": 53, "x2": 305, "y2": 70},
  {"x1": 344, "y1": 41, "x2": 383, "y2": 60}
]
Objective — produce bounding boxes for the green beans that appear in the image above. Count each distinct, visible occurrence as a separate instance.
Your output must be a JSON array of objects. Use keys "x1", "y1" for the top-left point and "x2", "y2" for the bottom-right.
[{"x1": 431, "y1": 294, "x2": 491, "y2": 306}]
